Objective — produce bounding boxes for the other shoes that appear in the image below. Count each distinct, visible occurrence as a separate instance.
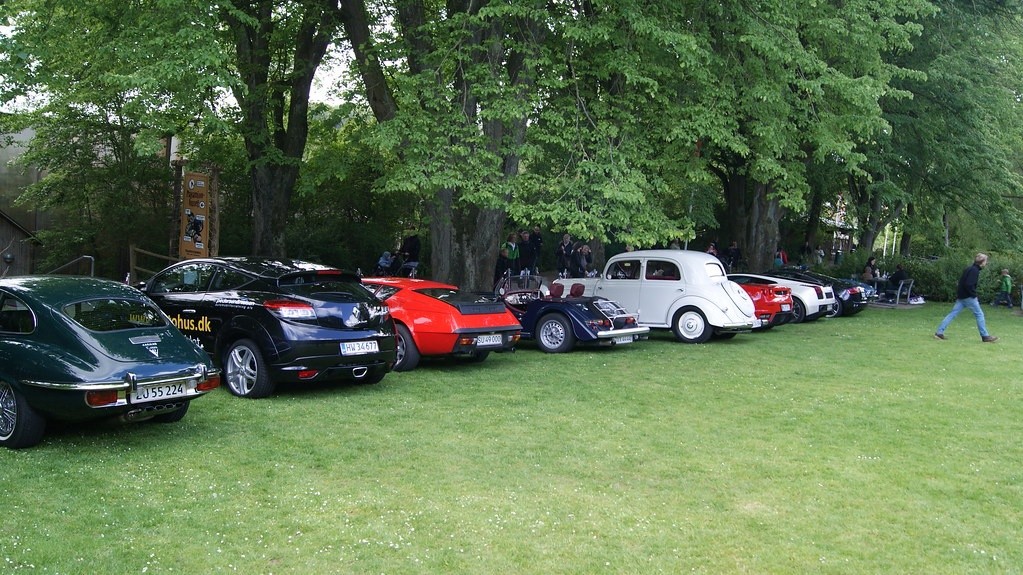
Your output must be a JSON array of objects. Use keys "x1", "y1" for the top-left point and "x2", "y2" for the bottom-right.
[
  {"x1": 886, "y1": 299, "x2": 896, "y2": 304},
  {"x1": 982, "y1": 336, "x2": 1000, "y2": 343},
  {"x1": 934, "y1": 332, "x2": 944, "y2": 339}
]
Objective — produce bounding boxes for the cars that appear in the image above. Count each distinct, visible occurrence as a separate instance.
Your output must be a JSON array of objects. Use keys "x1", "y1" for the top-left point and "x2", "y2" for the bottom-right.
[
  {"x1": 551, "y1": 249, "x2": 763, "y2": 343},
  {"x1": 727, "y1": 273, "x2": 835, "y2": 324},
  {"x1": 646, "y1": 275, "x2": 794, "y2": 332},
  {"x1": 130, "y1": 256, "x2": 398, "y2": 399},
  {"x1": 0, "y1": 273, "x2": 222, "y2": 449},
  {"x1": 359, "y1": 278, "x2": 523, "y2": 372},
  {"x1": 477, "y1": 275, "x2": 649, "y2": 354},
  {"x1": 764, "y1": 268, "x2": 878, "y2": 318}
]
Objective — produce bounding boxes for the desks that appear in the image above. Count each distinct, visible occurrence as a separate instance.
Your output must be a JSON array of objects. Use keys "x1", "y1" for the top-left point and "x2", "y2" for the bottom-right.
[{"x1": 867, "y1": 278, "x2": 889, "y2": 304}]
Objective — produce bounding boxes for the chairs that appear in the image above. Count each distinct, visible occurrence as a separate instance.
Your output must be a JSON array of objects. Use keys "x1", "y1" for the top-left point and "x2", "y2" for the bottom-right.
[
  {"x1": 566, "y1": 283, "x2": 585, "y2": 298},
  {"x1": 885, "y1": 278, "x2": 914, "y2": 305},
  {"x1": 545, "y1": 283, "x2": 564, "y2": 298},
  {"x1": 851, "y1": 273, "x2": 864, "y2": 283}
]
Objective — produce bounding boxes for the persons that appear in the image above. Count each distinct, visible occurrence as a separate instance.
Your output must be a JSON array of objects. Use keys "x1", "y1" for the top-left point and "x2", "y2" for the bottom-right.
[
  {"x1": 379, "y1": 224, "x2": 591, "y2": 287},
  {"x1": 625, "y1": 240, "x2": 910, "y2": 305},
  {"x1": 933, "y1": 253, "x2": 1000, "y2": 343},
  {"x1": 991, "y1": 269, "x2": 1013, "y2": 308}
]
